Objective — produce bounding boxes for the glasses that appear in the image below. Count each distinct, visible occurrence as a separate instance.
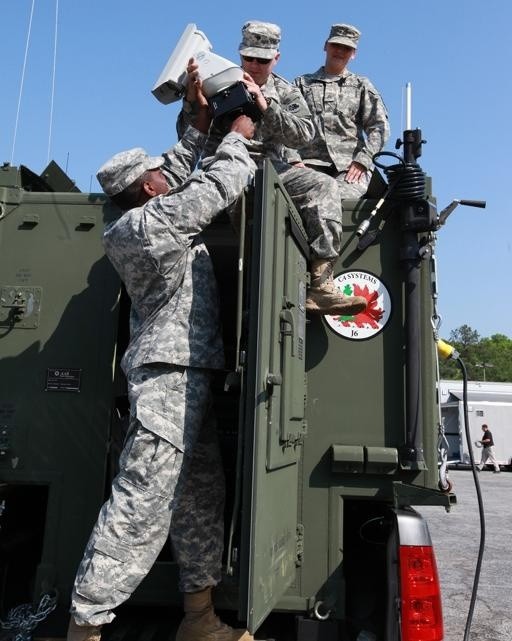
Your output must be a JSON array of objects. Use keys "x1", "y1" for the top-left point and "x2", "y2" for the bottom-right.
[{"x1": 243, "y1": 57, "x2": 271, "y2": 64}]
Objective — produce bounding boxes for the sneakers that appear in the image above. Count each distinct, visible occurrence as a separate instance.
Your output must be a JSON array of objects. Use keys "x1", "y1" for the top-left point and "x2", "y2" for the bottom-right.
[{"x1": 476, "y1": 466, "x2": 501, "y2": 473}]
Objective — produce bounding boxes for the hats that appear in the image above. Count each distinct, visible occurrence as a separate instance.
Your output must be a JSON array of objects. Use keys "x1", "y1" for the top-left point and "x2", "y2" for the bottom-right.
[
  {"x1": 239, "y1": 20, "x2": 281, "y2": 60},
  {"x1": 328, "y1": 24, "x2": 361, "y2": 49},
  {"x1": 95, "y1": 147, "x2": 165, "y2": 197}
]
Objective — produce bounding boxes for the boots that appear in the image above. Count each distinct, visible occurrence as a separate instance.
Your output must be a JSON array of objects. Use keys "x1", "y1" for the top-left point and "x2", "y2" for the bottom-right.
[
  {"x1": 306, "y1": 260, "x2": 368, "y2": 316},
  {"x1": 176, "y1": 586, "x2": 254, "y2": 641},
  {"x1": 67, "y1": 616, "x2": 101, "y2": 641}
]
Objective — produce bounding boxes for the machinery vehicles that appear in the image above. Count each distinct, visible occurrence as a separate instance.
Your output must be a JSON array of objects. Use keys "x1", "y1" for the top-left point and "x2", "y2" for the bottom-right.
[{"x1": 0, "y1": 126, "x2": 485, "y2": 641}]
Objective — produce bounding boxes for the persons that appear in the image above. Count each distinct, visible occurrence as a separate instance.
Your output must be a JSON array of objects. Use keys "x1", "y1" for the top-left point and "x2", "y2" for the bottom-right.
[
  {"x1": 284, "y1": 23, "x2": 391, "y2": 199},
  {"x1": 176, "y1": 20, "x2": 368, "y2": 316},
  {"x1": 475, "y1": 424, "x2": 500, "y2": 474},
  {"x1": 67, "y1": 78, "x2": 255, "y2": 641}
]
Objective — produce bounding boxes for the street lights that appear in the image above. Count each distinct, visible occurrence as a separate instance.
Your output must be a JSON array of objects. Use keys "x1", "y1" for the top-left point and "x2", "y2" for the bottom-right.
[{"x1": 471, "y1": 360, "x2": 493, "y2": 381}]
